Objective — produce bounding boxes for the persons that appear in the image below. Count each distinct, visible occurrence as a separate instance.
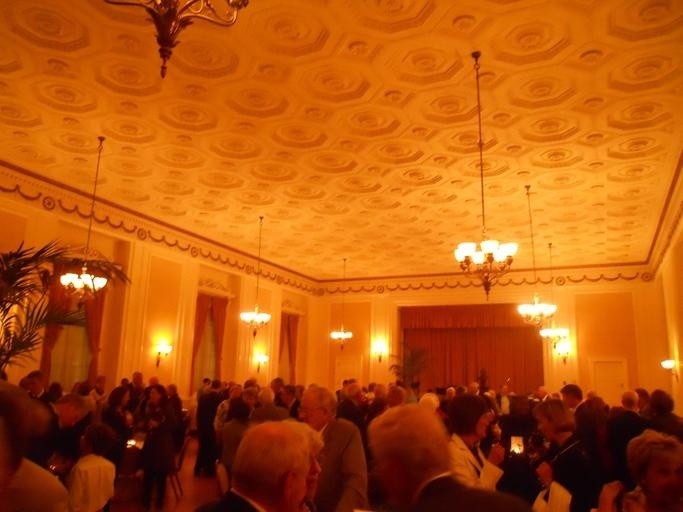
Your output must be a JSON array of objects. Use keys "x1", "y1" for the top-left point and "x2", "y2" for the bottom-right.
[
  {"x1": 190, "y1": 378, "x2": 682, "y2": 512},
  {"x1": 1, "y1": 369, "x2": 183, "y2": 512}
]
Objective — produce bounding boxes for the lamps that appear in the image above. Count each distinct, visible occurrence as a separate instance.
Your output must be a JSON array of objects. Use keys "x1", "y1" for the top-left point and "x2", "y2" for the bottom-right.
[
  {"x1": 253, "y1": 341, "x2": 269, "y2": 374},
  {"x1": 104, "y1": 0, "x2": 250, "y2": 77},
  {"x1": 657, "y1": 356, "x2": 679, "y2": 380},
  {"x1": 515, "y1": 180, "x2": 583, "y2": 370},
  {"x1": 57, "y1": 127, "x2": 110, "y2": 293},
  {"x1": 455, "y1": 51, "x2": 521, "y2": 302},
  {"x1": 239, "y1": 215, "x2": 274, "y2": 327},
  {"x1": 329, "y1": 255, "x2": 358, "y2": 341},
  {"x1": 148, "y1": 329, "x2": 173, "y2": 369},
  {"x1": 370, "y1": 338, "x2": 386, "y2": 361}
]
